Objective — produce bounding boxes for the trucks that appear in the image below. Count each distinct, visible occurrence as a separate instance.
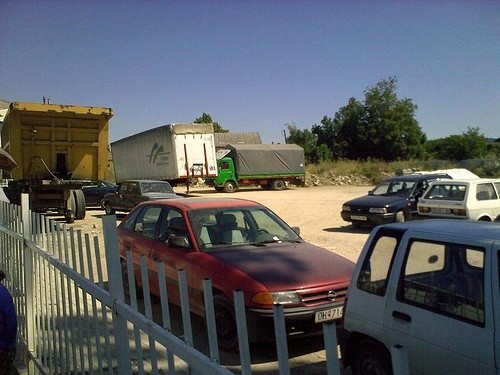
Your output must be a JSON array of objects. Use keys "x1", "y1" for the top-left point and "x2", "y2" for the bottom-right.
[
  {"x1": 215, "y1": 144, "x2": 306, "y2": 190},
  {"x1": 0, "y1": 101, "x2": 113, "y2": 223}
]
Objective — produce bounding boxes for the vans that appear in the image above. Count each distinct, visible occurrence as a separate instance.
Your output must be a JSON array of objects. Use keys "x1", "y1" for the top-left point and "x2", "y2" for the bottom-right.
[{"x1": 336, "y1": 218, "x2": 500, "y2": 375}]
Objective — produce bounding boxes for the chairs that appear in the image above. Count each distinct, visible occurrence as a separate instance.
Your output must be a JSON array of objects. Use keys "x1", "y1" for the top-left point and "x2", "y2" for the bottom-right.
[
  {"x1": 477, "y1": 191, "x2": 490, "y2": 199},
  {"x1": 170, "y1": 217, "x2": 205, "y2": 248},
  {"x1": 219, "y1": 215, "x2": 245, "y2": 244}
]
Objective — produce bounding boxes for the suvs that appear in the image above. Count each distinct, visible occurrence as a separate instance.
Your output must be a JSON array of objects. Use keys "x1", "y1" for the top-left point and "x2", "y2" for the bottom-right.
[{"x1": 417, "y1": 178, "x2": 500, "y2": 222}]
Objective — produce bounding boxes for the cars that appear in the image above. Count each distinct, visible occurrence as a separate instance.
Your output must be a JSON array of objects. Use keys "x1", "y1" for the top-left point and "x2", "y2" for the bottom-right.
[
  {"x1": 340, "y1": 171, "x2": 461, "y2": 228},
  {"x1": 84, "y1": 179, "x2": 121, "y2": 210},
  {"x1": 114, "y1": 197, "x2": 363, "y2": 350},
  {"x1": 101, "y1": 180, "x2": 186, "y2": 215}
]
